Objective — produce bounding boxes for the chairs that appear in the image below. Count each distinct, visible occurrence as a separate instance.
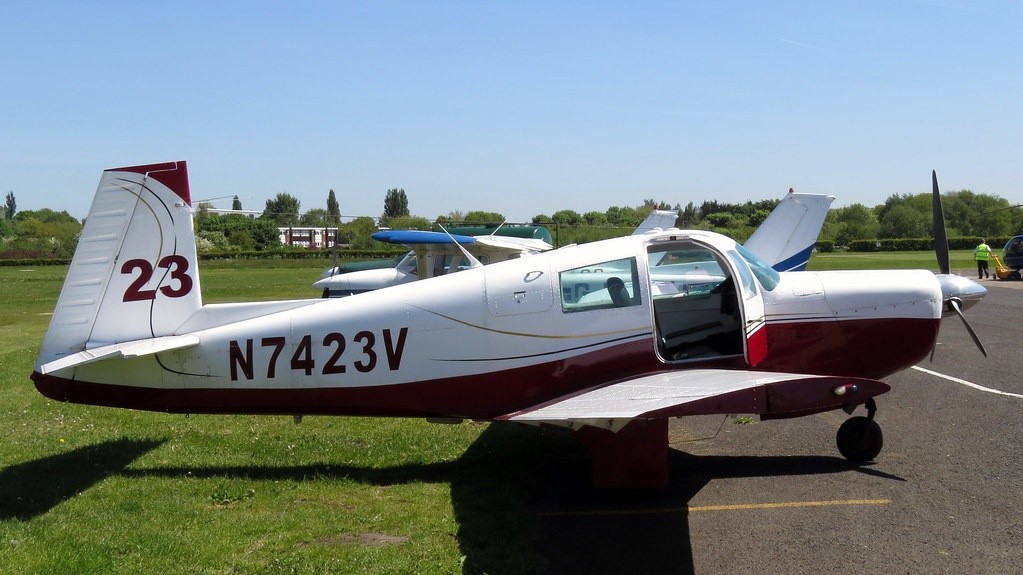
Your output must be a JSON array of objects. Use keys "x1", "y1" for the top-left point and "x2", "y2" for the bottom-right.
[{"x1": 606, "y1": 277, "x2": 694, "y2": 360}]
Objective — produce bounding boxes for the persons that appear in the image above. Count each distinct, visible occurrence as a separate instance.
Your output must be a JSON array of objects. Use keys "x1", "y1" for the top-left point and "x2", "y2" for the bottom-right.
[{"x1": 974, "y1": 239, "x2": 993, "y2": 280}]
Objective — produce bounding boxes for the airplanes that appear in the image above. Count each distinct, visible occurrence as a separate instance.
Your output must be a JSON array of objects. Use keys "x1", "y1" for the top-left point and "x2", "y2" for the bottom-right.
[
  {"x1": 307, "y1": 192, "x2": 833, "y2": 310},
  {"x1": 34, "y1": 158, "x2": 988, "y2": 470}
]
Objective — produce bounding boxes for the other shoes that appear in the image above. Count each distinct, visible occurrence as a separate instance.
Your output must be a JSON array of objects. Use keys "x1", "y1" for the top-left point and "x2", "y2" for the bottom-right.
[
  {"x1": 979, "y1": 278, "x2": 982, "y2": 279},
  {"x1": 986, "y1": 273, "x2": 988, "y2": 278}
]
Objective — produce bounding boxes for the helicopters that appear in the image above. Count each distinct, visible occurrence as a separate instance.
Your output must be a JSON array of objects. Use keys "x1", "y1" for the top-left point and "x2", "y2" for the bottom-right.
[{"x1": 975, "y1": 203, "x2": 1023, "y2": 280}]
